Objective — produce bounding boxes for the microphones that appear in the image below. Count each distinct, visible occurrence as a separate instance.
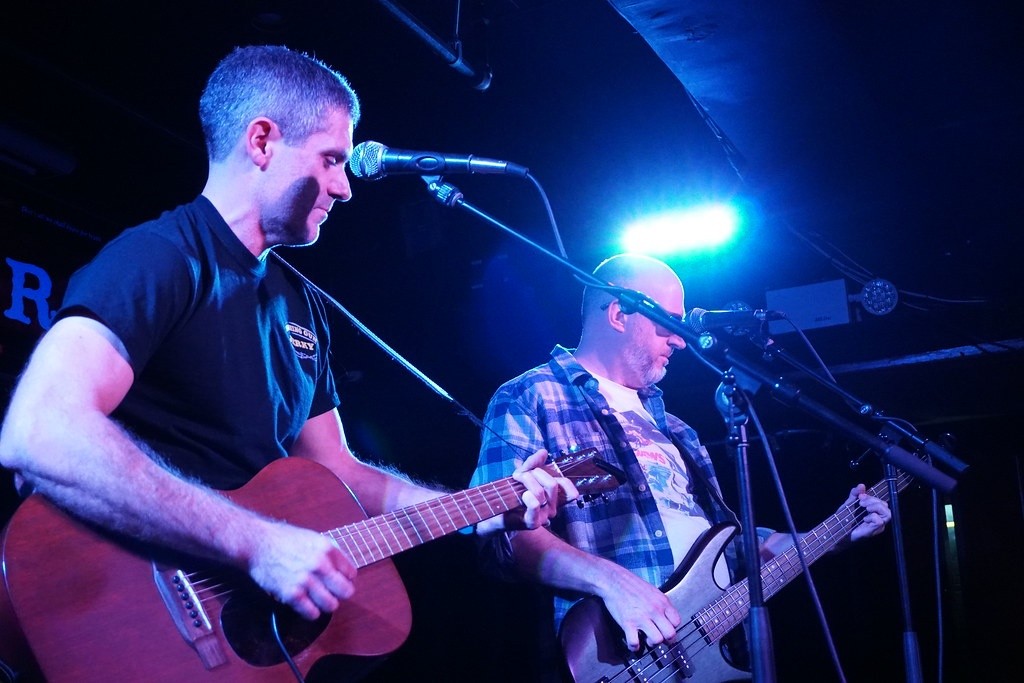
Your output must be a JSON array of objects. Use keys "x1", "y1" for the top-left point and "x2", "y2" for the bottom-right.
[
  {"x1": 349, "y1": 140, "x2": 529, "y2": 181},
  {"x1": 685, "y1": 308, "x2": 785, "y2": 333}
]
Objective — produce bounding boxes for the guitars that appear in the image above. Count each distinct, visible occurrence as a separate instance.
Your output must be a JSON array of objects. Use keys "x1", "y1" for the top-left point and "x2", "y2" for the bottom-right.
[
  {"x1": 558, "y1": 431, "x2": 955, "y2": 682},
  {"x1": 0, "y1": 447, "x2": 627, "y2": 683}
]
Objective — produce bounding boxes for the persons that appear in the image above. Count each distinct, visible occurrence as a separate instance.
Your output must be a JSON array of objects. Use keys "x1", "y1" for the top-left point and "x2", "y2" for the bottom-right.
[
  {"x1": 472, "y1": 255, "x2": 891, "y2": 683},
  {"x1": 0, "y1": 46, "x2": 580, "y2": 683}
]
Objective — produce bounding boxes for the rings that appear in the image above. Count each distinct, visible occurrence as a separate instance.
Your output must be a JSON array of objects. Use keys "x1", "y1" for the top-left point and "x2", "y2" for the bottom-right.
[{"x1": 541, "y1": 500, "x2": 548, "y2": 508}]
